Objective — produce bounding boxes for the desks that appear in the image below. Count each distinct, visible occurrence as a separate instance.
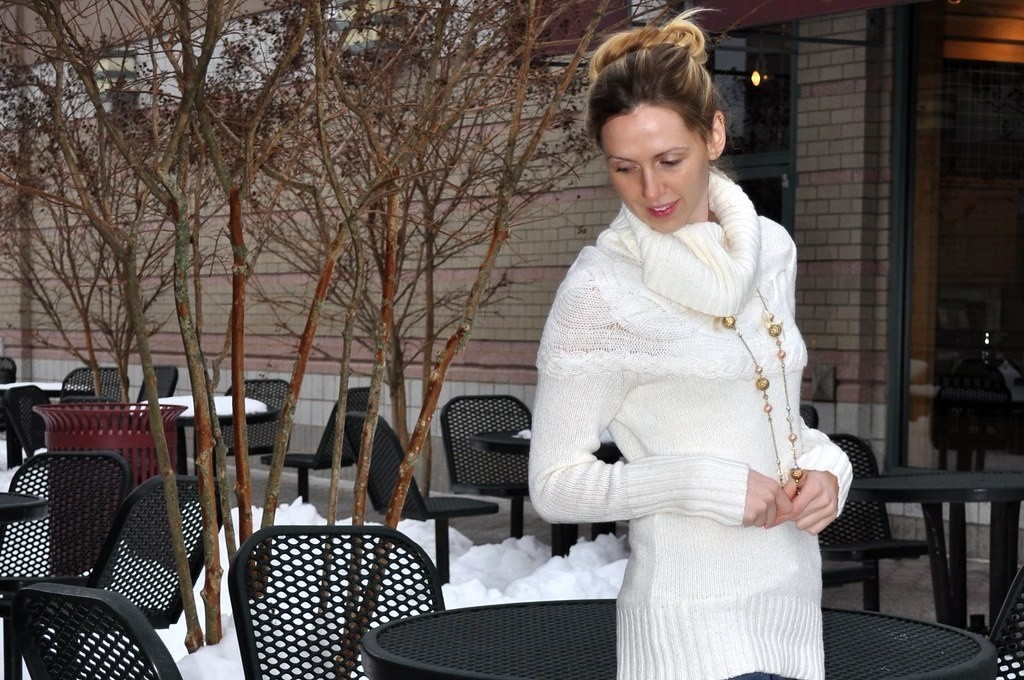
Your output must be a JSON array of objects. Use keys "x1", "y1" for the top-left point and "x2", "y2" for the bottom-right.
[
  {"x1": 0, "y1": 491, "x2": 48, "y2": 559},
  {"x1": 469, "y1": 430, "x2": 621, "y2": 558},
  {"x1": 1, "y1": 389, "x2": 96, "y2": 470},
  {"x1": 841, "y1": 472, "x2": 1023, "y2": 630},
  {"x1": 360, "y1": 599, "x2": 998, "y2": 680},
  {"x1": 128, "y1": 397, "x2": 280, "y2": 477}
]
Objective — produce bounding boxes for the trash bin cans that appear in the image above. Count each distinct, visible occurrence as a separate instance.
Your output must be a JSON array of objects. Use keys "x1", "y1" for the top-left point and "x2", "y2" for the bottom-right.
[{"x1": 32, "y1": 399, "x2": 190, "y2": 578}]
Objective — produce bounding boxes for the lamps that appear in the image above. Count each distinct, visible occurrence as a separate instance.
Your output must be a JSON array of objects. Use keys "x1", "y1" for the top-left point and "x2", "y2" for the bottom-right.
[{"x1": 751, "y1": 70, "x2": 776, "y2": 88}]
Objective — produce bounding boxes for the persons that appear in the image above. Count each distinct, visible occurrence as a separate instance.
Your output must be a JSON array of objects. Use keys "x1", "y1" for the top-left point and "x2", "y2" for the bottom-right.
[{"x1": 527, "y1": 21, "x2": 854, "y2": 680}]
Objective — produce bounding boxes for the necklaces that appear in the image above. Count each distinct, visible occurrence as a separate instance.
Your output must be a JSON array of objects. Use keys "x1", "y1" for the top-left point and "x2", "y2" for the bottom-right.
[{"x1": 724, "y1": 288, "x2": 801, "y2": 493}]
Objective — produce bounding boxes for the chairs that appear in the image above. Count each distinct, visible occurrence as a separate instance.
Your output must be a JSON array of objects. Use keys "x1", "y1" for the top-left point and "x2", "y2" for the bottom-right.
[{"x1": 0, "y1": 356, "x2": 1023, "y2": 680}]
